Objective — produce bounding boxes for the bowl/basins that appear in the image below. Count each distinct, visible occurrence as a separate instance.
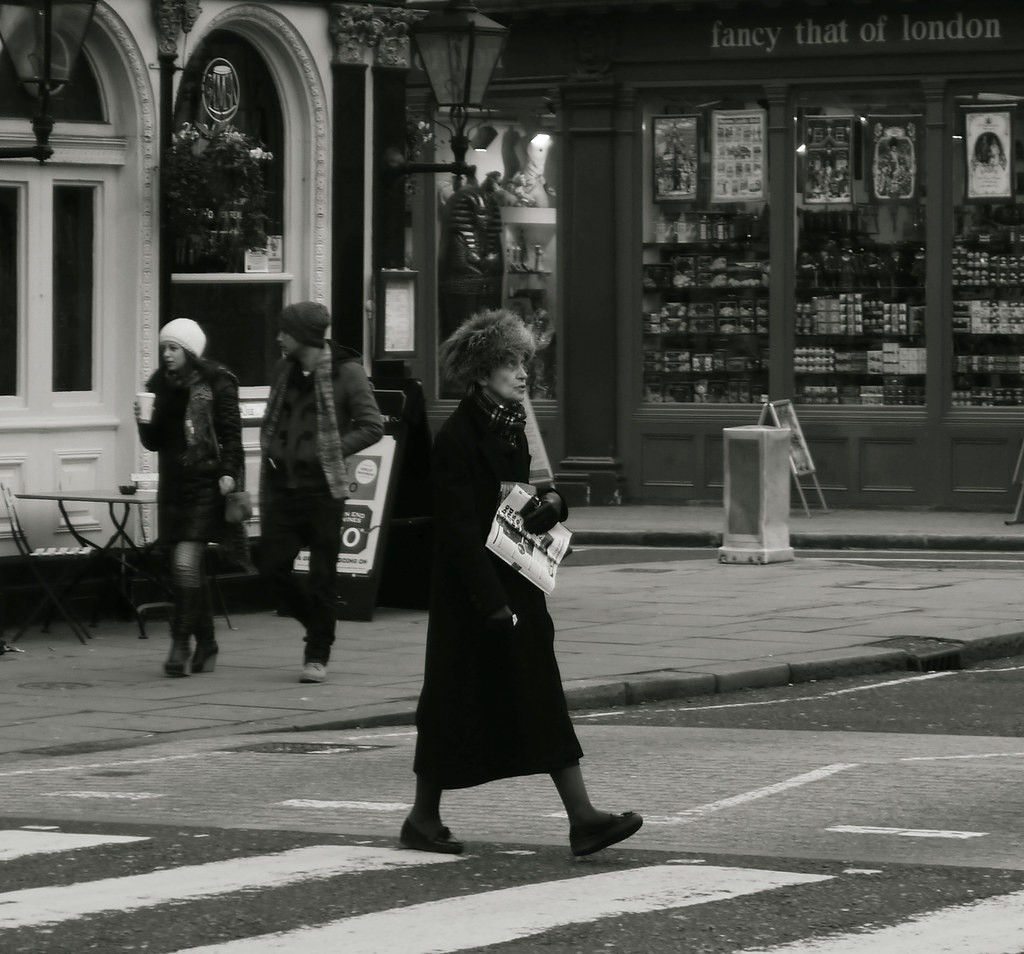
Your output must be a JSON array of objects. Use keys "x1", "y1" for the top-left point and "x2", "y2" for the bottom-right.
[{"x1": 119, "y1": 485, "x2": 137, "y2": 495}]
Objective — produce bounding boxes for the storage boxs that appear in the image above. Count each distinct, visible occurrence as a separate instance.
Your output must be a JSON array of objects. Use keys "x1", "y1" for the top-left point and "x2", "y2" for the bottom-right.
[{"x1": 642, "y1": 293, "x2": 1024, "y2": 408}]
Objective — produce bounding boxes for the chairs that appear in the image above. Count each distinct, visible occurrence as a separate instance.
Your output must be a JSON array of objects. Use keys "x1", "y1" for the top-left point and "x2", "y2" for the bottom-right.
[{"x1": 0, "y1": 481, "x2": 233, "y2": 646}]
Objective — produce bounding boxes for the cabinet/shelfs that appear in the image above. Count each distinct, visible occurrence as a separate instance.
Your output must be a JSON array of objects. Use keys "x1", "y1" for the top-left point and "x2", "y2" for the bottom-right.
[
  {"x1": 641, "y1": 209, "x2": 1024, "y2": 408},
  {"x1": 500, "y1": 206, "x2": 558, "y2": 402}
]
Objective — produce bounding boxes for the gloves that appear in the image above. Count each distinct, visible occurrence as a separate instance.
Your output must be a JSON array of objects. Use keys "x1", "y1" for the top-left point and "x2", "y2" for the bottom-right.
[
  {"x1": 487, "y1": 608, "x2": 514, "y2": 635},
  {"x1": 521, "y1": 490, "x2": 563, "y2": 535}
]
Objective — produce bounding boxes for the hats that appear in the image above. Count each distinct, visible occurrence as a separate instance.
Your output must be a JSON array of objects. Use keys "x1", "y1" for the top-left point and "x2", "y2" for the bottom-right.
[
  {"x1": 442, "y1": 308, "x2": 536, "y2": 385},
  {"x1": 277, "y1": 300, "x2": 330, "y2": 348},
  {"x1": 160, "y1": 318, "x2": 207, "y2": 359}
]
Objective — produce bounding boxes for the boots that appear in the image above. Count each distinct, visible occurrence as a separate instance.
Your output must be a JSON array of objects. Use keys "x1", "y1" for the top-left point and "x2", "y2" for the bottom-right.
[
  {"x1": 190, "y1": 583, "x2": 219, "y2": 672},
  {"x1": 164, "y1": 585, "x2": 191, "y2": 677}
]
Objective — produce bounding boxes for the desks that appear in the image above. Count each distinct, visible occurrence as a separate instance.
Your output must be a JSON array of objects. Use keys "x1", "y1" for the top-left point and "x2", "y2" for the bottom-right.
[{"x1": 13, "y1": 488, "x2": 158, "y2": 641}]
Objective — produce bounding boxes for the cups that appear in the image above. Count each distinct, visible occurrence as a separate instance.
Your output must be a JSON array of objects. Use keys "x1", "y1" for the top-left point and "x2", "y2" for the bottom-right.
[
  {"x1": 650, "y1": 212, "x2": 760, "y2": 244},
  {"x1": 137, "y1": 392, "x2": 157, "y2": 424}
]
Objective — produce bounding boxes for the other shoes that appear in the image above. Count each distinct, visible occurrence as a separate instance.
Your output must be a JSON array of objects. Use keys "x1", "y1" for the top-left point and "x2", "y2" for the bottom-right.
[
  {"x1": 398, "y1": 816, "x2": 463, "y2": 855},
  {"x1": 300, "y1": 660, "x2": 325, "y2": 682},
  {"x1": 570, "y1": 812, "x2": 643, "y2": 856}
]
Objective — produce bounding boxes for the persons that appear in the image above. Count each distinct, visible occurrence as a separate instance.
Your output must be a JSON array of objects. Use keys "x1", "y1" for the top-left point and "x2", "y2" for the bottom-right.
[
  {"x1": 133, "y1": 318, "x2": 245, "y2": 674},
  {"x1": 256, "y1": 301, "x2": 384, "y2": 682},
  {"x1": 400, "y1": 308, "x2": 644, "y2": 854}
]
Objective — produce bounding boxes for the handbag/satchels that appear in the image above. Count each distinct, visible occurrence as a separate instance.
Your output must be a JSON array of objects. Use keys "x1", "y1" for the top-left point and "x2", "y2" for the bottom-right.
[{"x1": 224, "y1": 491, "x2": 254, "y2": 522}]
